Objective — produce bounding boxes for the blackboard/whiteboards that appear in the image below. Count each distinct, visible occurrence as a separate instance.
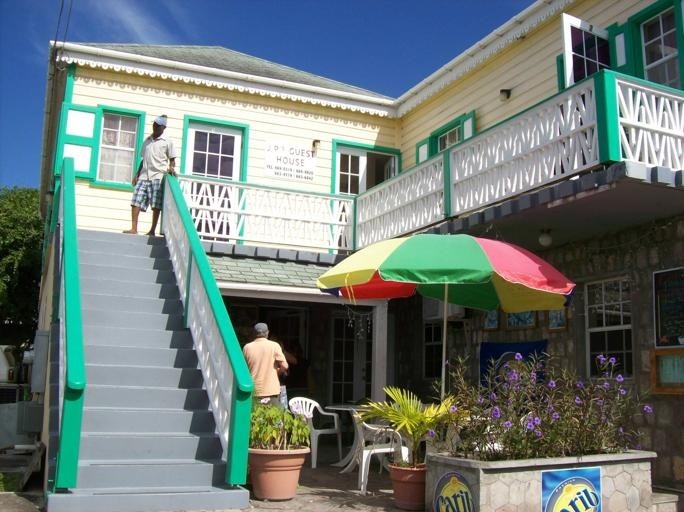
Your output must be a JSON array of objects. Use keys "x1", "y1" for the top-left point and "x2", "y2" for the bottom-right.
[{"x1": 653, "y1": 267, "x2": 684, "y2": 348}]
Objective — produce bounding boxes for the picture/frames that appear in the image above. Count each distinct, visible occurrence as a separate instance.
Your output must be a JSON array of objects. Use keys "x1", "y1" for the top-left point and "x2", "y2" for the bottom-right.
[
  {"x1": 479, "y1": 310, "x2": 500, "y2": 332},
  {"x1": 545, "y1": 308, "x2": 569, "y2": 333},
  {"x1": 505, "y1": 310, "x2": 539, "y2": 332}
]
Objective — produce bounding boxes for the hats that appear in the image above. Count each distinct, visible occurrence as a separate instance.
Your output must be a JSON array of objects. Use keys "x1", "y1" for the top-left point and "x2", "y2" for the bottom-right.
[
  {"x1": 254, "y1": 323, "x2": 268, "y2": 334},
  {"x1": 155, "y1": 116, "x2": 167, "y2": 127}
]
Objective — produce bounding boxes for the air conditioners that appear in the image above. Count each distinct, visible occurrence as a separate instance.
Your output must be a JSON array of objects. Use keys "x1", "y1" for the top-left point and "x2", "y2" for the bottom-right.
[{"x1": 421, "y1": 295, "x2": 466, "y2": 323}]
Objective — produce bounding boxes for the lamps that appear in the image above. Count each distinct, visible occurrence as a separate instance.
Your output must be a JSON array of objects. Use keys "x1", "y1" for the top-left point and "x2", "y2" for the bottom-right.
[
  {"x1": 313, "y1": 139, "x2": 321, "y2": 149},
  {"x1": 538, "y1": 228, "x2": 553, "y2": 246},
  {"x1": 499, "y1": 88, "x2": 511, "y2": 102}
]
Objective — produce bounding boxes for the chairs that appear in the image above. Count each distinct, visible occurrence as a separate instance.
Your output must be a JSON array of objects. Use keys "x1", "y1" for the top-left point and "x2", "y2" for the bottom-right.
[
  {"x1": 288, "y1": 396, "x2": 342, "y2": 469},
  {"x1": 348, "y1": 407, "x2": 409, "y2": 496},
  {"x1": 378, "y1": 399, "x2": 426, "y2": 475}
]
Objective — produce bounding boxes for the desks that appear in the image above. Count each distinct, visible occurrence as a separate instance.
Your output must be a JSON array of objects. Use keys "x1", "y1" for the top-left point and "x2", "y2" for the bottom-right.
[{"x1": 324, "y1": 405, "x2": 386, "y2": 475}]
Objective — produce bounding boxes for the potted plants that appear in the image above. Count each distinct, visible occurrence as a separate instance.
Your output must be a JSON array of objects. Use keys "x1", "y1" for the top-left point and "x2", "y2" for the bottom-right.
[
  {"x1": 354, "y1": 385, "x2": 472, "y2": 511},
  {"x1": 248, "y1": 404, "x2": 311, "y2": 501}
]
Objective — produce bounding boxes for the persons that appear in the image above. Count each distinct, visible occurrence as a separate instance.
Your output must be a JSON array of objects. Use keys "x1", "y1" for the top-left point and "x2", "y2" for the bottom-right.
[
  {"x1": 264, "y1": 332, "x2": 297, "y2": 408},
  {"x1": 118, "y1": 114, "x2": 178, "y2": 236},
  {"x1": 242, "y1": 322, "x2": 287, "y2": 405}
]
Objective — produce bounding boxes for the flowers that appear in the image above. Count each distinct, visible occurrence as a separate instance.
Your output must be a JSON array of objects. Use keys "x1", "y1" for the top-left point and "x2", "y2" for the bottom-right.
[{"x1": 430, "y1": 336, "x2": 656, "y2": 462}]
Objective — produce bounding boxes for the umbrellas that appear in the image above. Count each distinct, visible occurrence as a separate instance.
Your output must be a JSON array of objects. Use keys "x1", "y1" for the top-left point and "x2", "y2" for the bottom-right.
[{"x1": 313, "y1": 227, "x2": 580, "y2": 413}]
